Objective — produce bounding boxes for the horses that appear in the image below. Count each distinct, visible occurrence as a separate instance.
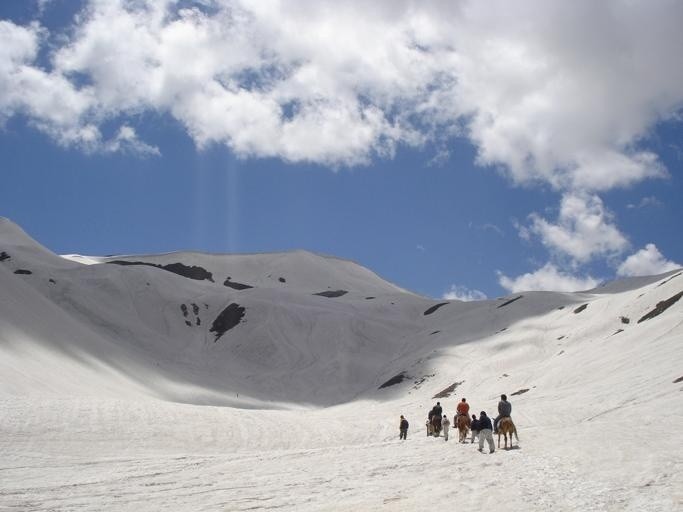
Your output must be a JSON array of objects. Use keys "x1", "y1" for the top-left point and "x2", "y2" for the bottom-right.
[
  {"x1": 492, "y1": 415, "x2": 521, "y2": 451},
  {"x1": 428, "y1": 413, "x2": 442, "y2": 437},
  {"x1": 456, "y1": 411, "x2": 471, "y2": 444}
]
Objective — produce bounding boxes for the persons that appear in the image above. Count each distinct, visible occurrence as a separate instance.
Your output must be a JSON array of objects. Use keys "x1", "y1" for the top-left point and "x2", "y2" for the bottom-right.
[
  {"x1": 427, "y1": 394, "x2": 512, "y2": 454},
  {"x1": 398, "y1": 416, "x2": 409, "y2": 439}
]
